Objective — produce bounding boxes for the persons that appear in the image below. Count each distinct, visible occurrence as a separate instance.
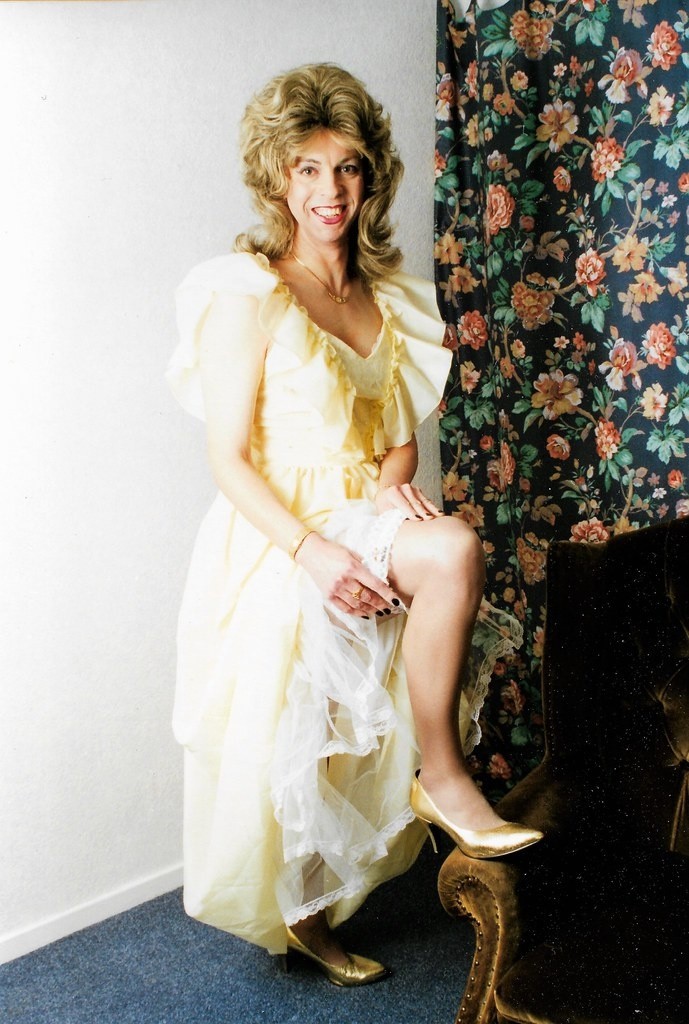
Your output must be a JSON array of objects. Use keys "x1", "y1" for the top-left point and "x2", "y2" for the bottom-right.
[{"x1": 171, "y1": 62, "x2": 545, "y2": 987}]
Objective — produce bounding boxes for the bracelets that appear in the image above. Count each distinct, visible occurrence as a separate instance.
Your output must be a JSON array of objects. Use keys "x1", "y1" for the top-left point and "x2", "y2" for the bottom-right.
[{"x1": 287, "y1": 526, "x2": 317, "y2": 559}]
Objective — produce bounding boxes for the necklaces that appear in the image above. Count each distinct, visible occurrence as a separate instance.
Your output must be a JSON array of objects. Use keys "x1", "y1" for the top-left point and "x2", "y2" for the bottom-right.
[{"x1": 290, "y1": 250, "x2": 354, "y2": 304}]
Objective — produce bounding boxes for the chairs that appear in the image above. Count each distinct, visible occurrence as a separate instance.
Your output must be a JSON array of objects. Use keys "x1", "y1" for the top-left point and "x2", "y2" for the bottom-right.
[{"x1": 438, "y1": 517, "x2": 689, "y2": 1024}]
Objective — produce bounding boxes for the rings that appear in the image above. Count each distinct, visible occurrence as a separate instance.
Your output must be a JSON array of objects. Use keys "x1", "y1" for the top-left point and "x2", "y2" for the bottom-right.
[{"x1": 353, "y1": 586, "x2": 365, "y2": 599}]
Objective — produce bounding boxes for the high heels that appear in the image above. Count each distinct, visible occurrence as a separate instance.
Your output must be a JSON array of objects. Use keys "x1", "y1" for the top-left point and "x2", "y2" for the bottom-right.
[
  {"x1": 279, "y1": 924, "x2": 385, "y2": 988},
  {"x1": 410, "y1": 769, "x2": 543, "y2": 858}
]
